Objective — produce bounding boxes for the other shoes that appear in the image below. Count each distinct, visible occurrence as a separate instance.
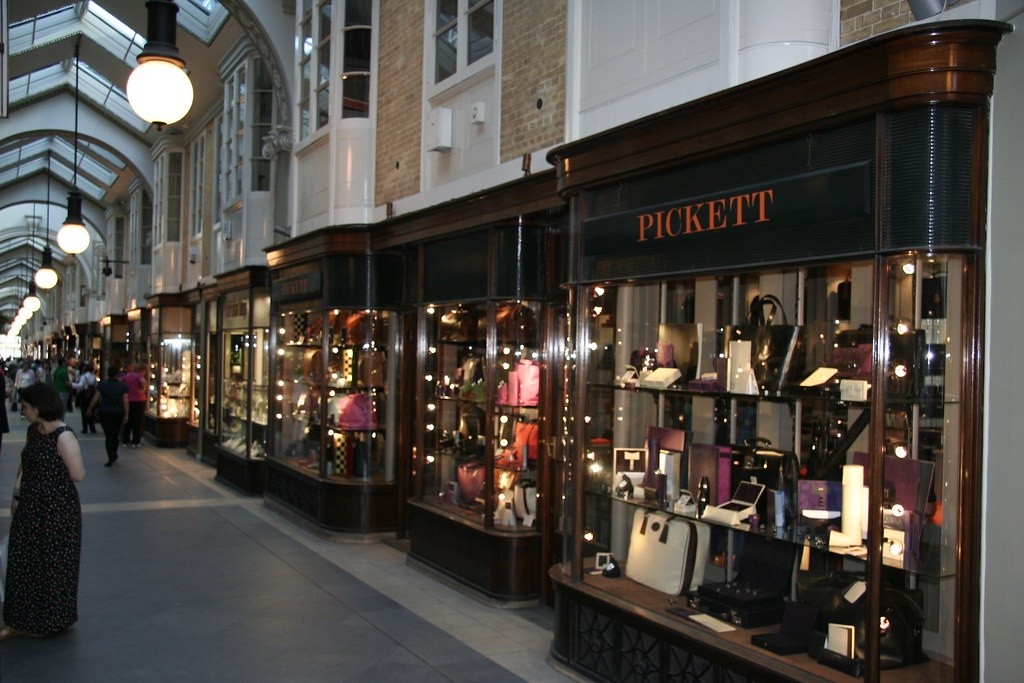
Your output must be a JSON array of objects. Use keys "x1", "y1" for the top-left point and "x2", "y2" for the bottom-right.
[
  {"x1": 122, "y1": 443, "x2": 131, "y2": 447},
  {"x1": 132, "y1": 443, "x2": 145, "y2": 448},
  {"x1": 21, "y1": 415, "x2": 27, "y2": 419},
  {"x1": 0, "y1": 625, "x2": 26, "y2": 641}
]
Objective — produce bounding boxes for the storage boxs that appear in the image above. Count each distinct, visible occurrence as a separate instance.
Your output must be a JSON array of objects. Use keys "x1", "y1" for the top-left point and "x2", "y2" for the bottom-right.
[
  {"x1": 673, "y1": 489, "x2": 697, "y2": 514},
  {"x1": 716, "y1": 480, "x2": 766, "y2": 520},
  {"x1": 645, "y1": 367, "x2": 682, "y2": 388}
]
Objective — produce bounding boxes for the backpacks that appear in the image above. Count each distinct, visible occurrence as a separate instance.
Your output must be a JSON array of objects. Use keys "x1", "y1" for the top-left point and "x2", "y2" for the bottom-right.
[{"x1": 85, "y1": 376, "x2": 98, "y2": 407}]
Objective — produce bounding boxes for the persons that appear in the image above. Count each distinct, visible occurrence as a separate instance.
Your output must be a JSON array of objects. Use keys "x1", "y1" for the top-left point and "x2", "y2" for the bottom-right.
[
  {"x1": 0, "y1": 384, "x2": 86, "y2": 643},
  {"x1": 85, "y1": 365, "x2": 129, "y2": 467},
  {"x1": 70, "y1": 362, "x2": 97, "y2": 434},
  {"x1": 0, "y1": 367, "x2": 9, "y2": 452},
  {"x1": 52, "y1": 356, "x2": 72, "y2": 422},
  {"x1": 118, "y1": 362, "x2": 148, "y2": 449},
  {"x1": 0, "y1": 355, "x2": 127, "y2": 419}
]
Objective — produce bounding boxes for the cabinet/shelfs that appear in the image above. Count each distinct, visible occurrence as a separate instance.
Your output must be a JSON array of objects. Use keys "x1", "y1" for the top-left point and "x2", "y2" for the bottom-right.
[
  {"x1": 222, "y1": 378, "x2": 268, "y2": 457},
  {"x1": 431, "y1": 339, "x2": 540, "y2": 533},
  {"x1": 585, "y1": 381, "x2": 960, "y2": 683},
  {"x1": 285, "y1": 344, "x2": 387, "y2": 483}
]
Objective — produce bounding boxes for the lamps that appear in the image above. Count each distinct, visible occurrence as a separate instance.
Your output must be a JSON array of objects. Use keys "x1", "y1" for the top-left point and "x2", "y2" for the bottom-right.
[
  {"x1": 56, "y1": 50, "x2": 90, "y2": 257},
  {"x1": 23, "y1": 227, "x2": 41, "y2": 312},
  {"x1": 100, "y1": 256, "x2": 130, "y2": 277},
  {"x1": 34, "y1": 156, "x2": 58, "y2": 292},
  {"x1": 126, "y1": 0, "x2": 195, "y2": 132}
]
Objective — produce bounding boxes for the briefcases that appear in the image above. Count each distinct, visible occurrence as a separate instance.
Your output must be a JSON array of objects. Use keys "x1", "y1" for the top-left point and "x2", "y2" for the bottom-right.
[
  {"x1": 725, "y1": 437, "x2": 798, "y2": 526},
  {"x1": 687, "y1": 535, "x2": 794, "y2": 628},
  {"x1": 837, "y1": 322, "x2": 926, "y2": 398}
]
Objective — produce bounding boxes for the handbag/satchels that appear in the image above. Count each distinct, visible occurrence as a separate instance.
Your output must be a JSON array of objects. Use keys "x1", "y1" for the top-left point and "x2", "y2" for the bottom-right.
[
  {"x1": 10, "y1": 390, "x2": 17, "y2": 411},
  {"x1": 13, "y1": 465, "x2": 23, "y2": 500},
  {"x1": 796, "y1": 569, "x2": 923, "y2": 677},
  {"x1": 443, "y1": 408, "x2": 539, "y2": 527},
  {"x1": 629, "y1": 347, "x2": 657, "y2": 378},
  {"x1": 75, "y1": 390, "x2": 89, "y2": 409},
  {"x1": 625, "y1": 507, "x2": 710, "y2": 594},
  {"x1": 287, "y1": 311, "x2": 386, "y2": 474},
  {"x1": 5, "y1": 376, "x2": 14, "y2": 394},
  {"x1": 823, "y1": 346, "x2": 868, "y2": 376},
  {"x1": 725, "y1": 295, "x2": 804, "y2": 403},
  {"x1": 0, "y1": 534, "x2": 9, "y2": 602},
  {"x1": 441, "y1": 305, "x2": 538, "y2": 407}
]
